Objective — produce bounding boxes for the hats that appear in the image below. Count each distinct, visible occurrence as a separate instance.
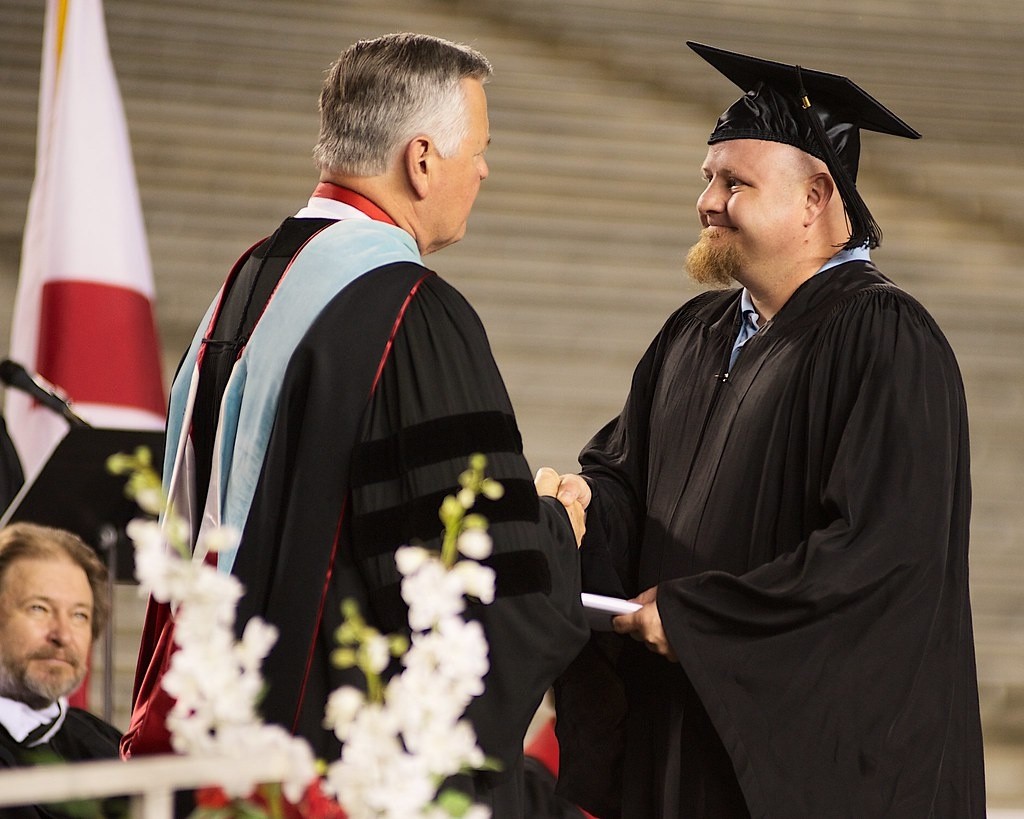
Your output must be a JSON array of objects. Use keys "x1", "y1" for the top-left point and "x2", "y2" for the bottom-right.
[{"x1": 685, "y1": 38, "x2": 921, "y2": 249}]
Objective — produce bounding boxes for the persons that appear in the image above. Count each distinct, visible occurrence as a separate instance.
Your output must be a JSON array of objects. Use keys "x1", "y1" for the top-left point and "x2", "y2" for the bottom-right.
[
  {"x1": 523, "y1": 33, "x2": 989, "y2": 819},
  {"x1": 123, "y1": 30, "x2": 588, "y2": 819},
  {"x1": 0, "y1": 525, "x2": 200, "y2": 818}
]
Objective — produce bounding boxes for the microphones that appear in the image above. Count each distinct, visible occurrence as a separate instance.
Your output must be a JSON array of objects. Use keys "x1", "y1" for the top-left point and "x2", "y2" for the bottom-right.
[{"x1": 0, "y1": 360, "x2": 67, "y2": 415}]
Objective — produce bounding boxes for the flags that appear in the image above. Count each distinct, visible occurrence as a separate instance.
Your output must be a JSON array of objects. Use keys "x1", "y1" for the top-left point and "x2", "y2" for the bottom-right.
[{"x1": 2, "y1": 1, "x2": 168, "y2": 482}]
